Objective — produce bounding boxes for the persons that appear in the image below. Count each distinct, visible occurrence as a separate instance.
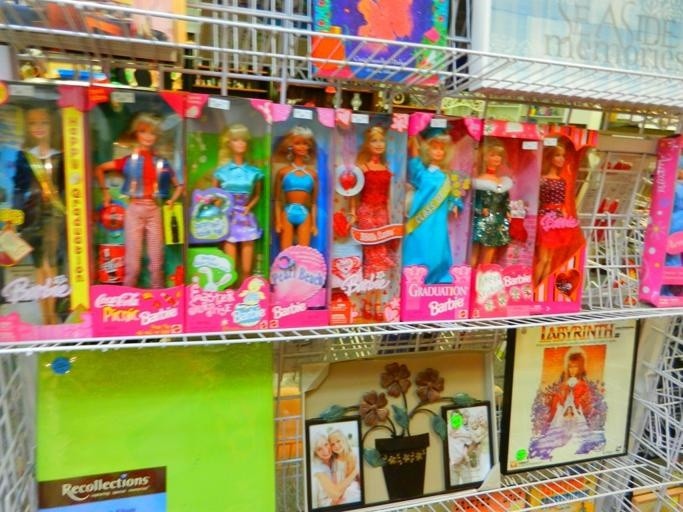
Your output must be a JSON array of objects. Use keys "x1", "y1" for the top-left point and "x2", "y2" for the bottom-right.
[
  {"x1": 311, "y1": 428, "x2": 361, "y2": 506},
  {"x1": 9, "y1": 105, "x2": 64, "y2": 323},
  {"x1": 533, "y1": 142, "x2": 581, "y2": 285},
  {"x1": 212, "y1": 120, "x2": 265, "y2": 281},
  {"x1": 271, "y1": 124, "x2": 316, "y2": 249},
  {"x1": 449, "y1": 409, "x2": 488, "y2": 484},
  {"x1": 548, "y1": 353, "x2": 594, "y2": 419},
  {"x1": 93, "y1": 111, "x2": 182, "y2": 287},
  {"x1": 470, "y1": 138, "x2": 515, "y2": 267},
  {"x1": 402, "y1": 129, "x2": 463, "y2": 285},
  {"x1": 660, "y1": 155, "x2": 683, "y2": 296},
  {"x1": 349, "y1": 126, "x2": 395, "y2": 322}
]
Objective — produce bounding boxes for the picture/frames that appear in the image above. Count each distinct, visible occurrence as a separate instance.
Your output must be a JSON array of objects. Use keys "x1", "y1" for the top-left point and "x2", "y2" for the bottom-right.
[
  {"x1": 439, "y1": 401, "x2": 494, "y2": 491},
  {"x1": 303, "y1": 414, "x2": 366, "y2": 512}
]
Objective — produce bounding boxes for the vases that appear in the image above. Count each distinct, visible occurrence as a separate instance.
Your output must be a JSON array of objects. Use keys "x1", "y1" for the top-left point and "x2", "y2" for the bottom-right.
[{"x1": 370, "y1": 433, "x2": 429, "y2": 504}]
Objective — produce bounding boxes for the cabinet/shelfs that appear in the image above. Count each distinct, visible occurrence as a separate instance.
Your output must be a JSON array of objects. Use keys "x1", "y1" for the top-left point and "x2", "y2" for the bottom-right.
[{"x1": 1, "y1": 0, "x2": 682, "y2": 511}]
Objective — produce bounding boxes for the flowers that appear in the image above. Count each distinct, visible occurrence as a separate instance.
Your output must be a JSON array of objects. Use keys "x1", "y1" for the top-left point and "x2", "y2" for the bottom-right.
[{"x1": 318, "y1": 361, "x2": 482, "y2": 471}]
